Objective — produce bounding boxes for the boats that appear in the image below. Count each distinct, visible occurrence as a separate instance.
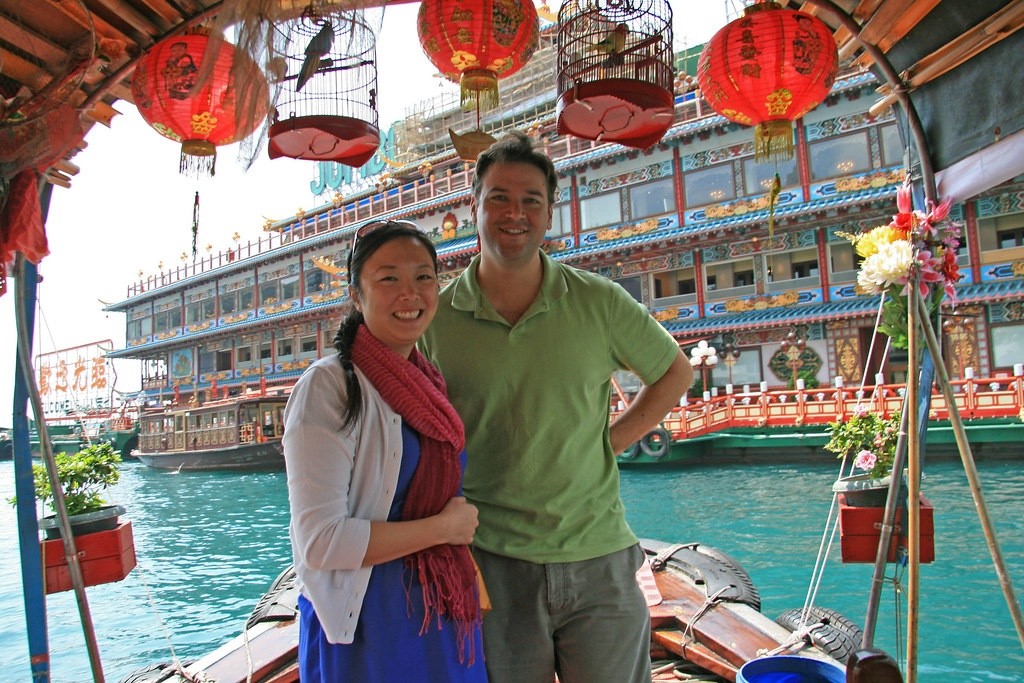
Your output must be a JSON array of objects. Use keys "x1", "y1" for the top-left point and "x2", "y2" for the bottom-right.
[{"x1": 129, "y1": 392, "x2": 294, "y2": 472}]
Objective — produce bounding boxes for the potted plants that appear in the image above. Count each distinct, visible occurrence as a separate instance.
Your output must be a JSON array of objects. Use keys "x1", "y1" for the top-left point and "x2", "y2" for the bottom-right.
[{"x1": 6, "y1": 441, "x2": 127, "y2": 540}]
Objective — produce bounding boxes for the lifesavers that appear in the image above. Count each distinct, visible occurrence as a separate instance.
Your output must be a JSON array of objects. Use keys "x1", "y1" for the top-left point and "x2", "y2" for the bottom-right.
[{"x1": 617, "y1": 422, "x2": 672, "y2": 462}]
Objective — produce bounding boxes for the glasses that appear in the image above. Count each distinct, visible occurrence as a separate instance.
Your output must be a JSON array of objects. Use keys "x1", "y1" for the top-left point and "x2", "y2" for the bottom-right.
[{"x1": 349, "y1": 220, "x2": 427, "y2": 283}]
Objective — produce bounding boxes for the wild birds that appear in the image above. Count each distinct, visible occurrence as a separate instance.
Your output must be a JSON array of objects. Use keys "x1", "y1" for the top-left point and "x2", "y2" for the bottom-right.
[
  {"x1": 583, "y1": 23, "x2": 629, "y2": 56},
  {"x1": 296, "y1": 21, "x2": 334, "y2": 92}
]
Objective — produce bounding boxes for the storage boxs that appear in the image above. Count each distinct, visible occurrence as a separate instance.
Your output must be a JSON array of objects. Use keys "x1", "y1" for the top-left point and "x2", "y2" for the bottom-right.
[
  {"x1": 838, "y1": 492, "x2": 935, "y2": 563},
  {"x1": 41, "y1": 521, "x2": 137, "y2": 595}
]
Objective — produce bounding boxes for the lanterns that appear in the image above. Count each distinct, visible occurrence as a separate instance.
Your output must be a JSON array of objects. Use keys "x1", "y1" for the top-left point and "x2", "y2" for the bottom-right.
[
  {"x1": 697, "y1": 1, "x2": 840, "y2": 164},
  {"x1": 131, "y1": 25, "x2": 271, "y2": 177},
  {"x1": 416, "y1": 0, "x2": 540, "y2": 111}
]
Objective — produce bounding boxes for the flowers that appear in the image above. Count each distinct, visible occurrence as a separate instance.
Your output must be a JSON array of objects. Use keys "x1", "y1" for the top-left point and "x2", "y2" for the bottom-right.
[
  {"x1": 835, "y1": 172, "x2": 962, "y2": 352},
  {"x1": 823, "y1": 407, "x2": 904, "y2": 482}
]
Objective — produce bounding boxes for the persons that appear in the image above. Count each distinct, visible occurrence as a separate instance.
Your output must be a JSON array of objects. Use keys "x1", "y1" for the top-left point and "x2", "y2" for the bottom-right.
[
  {"x1": 416, "y1": 130, "x2": 696, "y2": 683},
  {"x1": 281, "y1": 220, "x2": 491, "y2": 683}
]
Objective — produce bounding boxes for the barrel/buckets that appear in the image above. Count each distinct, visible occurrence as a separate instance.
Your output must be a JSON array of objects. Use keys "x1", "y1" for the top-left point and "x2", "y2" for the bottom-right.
[{"x1": 735, "y1": 656, "x2": 847, "y2": 683}]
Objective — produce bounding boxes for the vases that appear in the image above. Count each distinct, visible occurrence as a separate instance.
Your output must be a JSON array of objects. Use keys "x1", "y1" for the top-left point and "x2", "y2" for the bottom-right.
[{"x1": 833, "y1": 469, "x2": 928, "y2": 504}]
